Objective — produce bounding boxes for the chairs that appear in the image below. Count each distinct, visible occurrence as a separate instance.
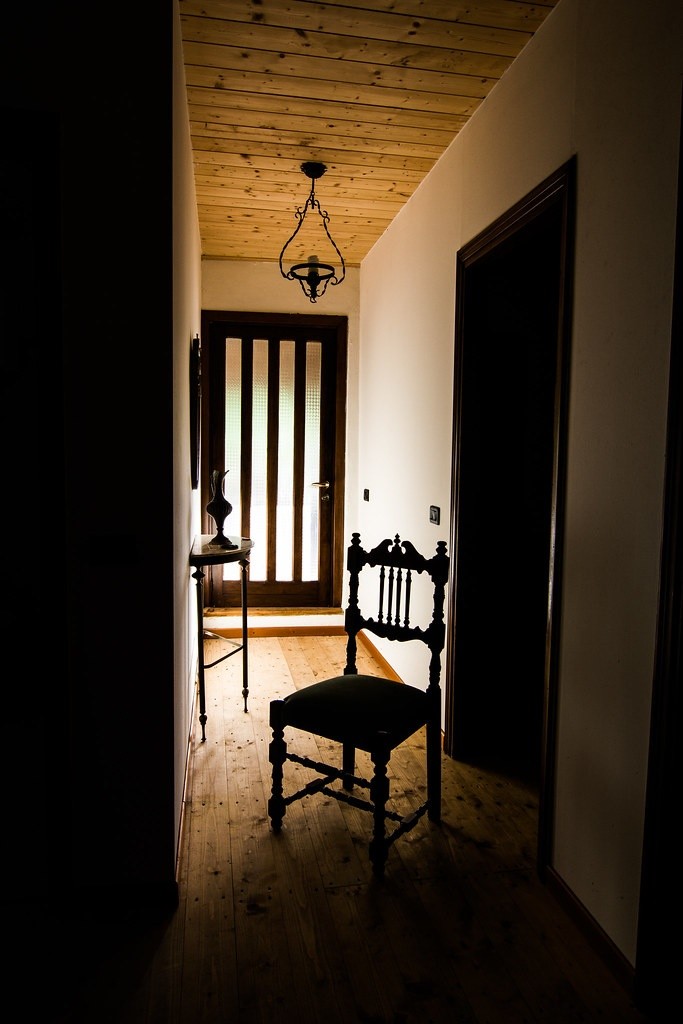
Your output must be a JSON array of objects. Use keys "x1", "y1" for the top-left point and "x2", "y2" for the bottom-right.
[{"x1": 268, "y1": 533, "x2": 450, "y2": 880}]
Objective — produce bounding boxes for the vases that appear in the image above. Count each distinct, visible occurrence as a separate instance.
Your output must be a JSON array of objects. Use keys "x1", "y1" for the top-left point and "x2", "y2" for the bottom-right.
[{"x1": 206, "y1": 470, "x2": 233, "y2": 545}]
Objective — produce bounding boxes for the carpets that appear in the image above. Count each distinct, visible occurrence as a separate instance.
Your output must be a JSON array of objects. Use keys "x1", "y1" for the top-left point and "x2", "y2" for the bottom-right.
[{"x1": 203, "y1": 604, "x2": 348, "y2": 639}]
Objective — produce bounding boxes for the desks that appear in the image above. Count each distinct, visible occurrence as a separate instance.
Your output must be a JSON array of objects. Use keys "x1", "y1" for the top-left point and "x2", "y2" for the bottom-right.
[{"x1": 188, "y1": 534, "x2": 255, "y2": 742}]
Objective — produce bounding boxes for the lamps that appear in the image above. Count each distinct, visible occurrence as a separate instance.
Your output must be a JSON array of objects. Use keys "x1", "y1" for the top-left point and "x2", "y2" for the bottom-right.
[{"x1": 279, "y1": 161, "x2": 346, "y2": 303}]
